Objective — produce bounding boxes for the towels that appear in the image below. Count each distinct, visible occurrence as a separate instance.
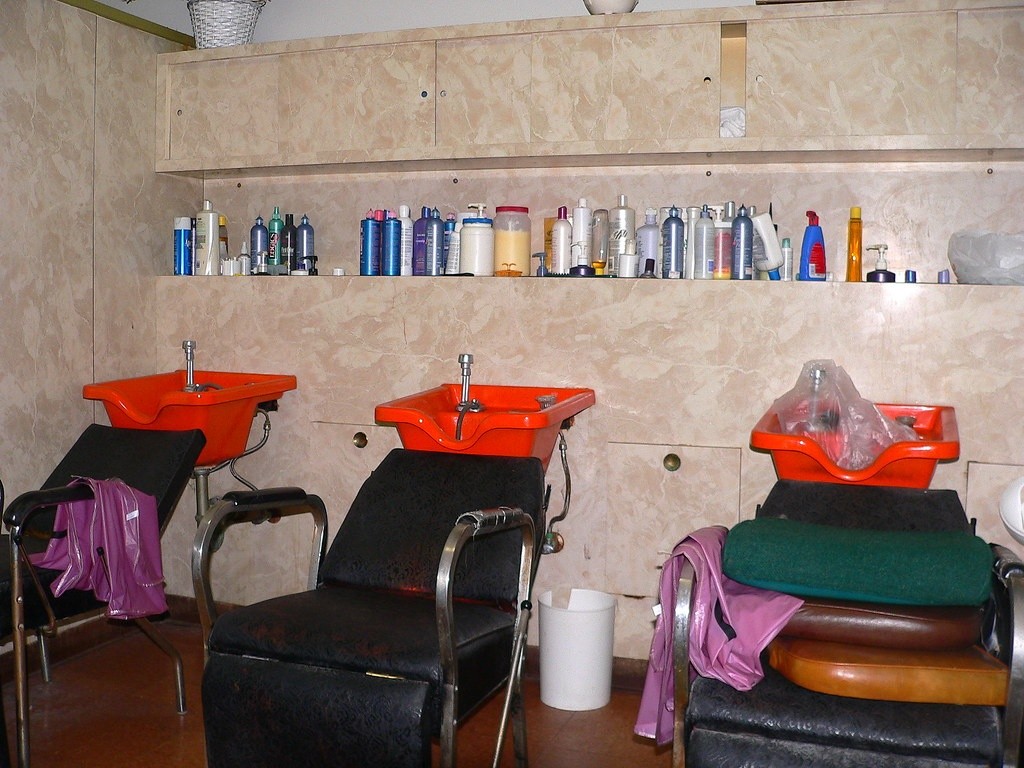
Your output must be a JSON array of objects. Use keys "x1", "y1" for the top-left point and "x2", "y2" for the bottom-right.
[{"x1": 718, "y1": 106, "x2": 746, "y2": 137}]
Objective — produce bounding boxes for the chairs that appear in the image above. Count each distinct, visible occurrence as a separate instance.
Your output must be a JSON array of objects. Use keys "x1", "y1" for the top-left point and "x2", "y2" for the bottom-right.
[
  {"x1": 672, "y1": 477, "x2": 1024, "y2": 768},
  {"x1": 0, "y1": 422, "x2": 207, "y2": 768},
  {"x1": 193, "y1": 452, "x2": 545, "y2": 768}
]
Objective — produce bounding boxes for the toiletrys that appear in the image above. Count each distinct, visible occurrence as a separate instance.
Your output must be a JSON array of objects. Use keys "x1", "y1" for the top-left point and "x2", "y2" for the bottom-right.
[
  {"x1": 845, "y1": 205, "x2": 951, "y2": 282},
  {"x1": 168, "y1": 200, "x2": 320, "y2": 275},
  {"x1": 359, "y1": 199, "x2": 826, "y2": 287}
]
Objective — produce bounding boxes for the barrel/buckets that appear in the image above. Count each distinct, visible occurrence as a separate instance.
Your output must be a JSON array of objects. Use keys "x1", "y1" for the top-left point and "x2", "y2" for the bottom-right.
[{"x1": 538, "y1": 589, "x2": 616, "y2": 711}]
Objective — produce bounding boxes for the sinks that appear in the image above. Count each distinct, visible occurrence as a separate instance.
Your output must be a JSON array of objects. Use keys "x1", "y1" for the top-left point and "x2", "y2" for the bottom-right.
[
  {"x1": 749, "y1": 397, "x2": 962, "y2": 490},
  {"x1": 82, "y1": 369, "x2": 296, "y2": 468},
  {"x1": 373, "y1": 384, "x2": 596, "y2": 478}
]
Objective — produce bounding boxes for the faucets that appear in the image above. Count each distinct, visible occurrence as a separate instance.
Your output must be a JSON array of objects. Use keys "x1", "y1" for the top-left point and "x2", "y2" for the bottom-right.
[
  {"x1": 805, "y1": 367, "x2": 828, "y2": 433},
  {"x1": 458, "y1": 354, "x2": 474, "y2": 406},
  {"x1": 182, "y1": 340, "x2": 197, "y2": 387}
]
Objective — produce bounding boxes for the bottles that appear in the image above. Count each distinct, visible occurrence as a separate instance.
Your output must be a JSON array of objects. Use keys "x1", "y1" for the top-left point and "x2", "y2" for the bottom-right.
[
  {"x1": 173, "y1": 216, "x2": 192, "y2": 275},
  {"x1": 219, "y1": 215, "x2": 228, "y2": 258}
]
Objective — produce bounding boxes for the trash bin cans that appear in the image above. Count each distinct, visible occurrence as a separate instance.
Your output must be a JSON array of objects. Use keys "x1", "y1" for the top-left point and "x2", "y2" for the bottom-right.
[{"x1": 537, "y1": 588, "x2": 616, "y2": 712}]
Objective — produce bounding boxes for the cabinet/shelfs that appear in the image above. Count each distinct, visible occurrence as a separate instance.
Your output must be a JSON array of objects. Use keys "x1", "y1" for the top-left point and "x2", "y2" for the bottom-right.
[{"x1": 157, "y1": 2, "x2": 1024, "y2": 178}]
